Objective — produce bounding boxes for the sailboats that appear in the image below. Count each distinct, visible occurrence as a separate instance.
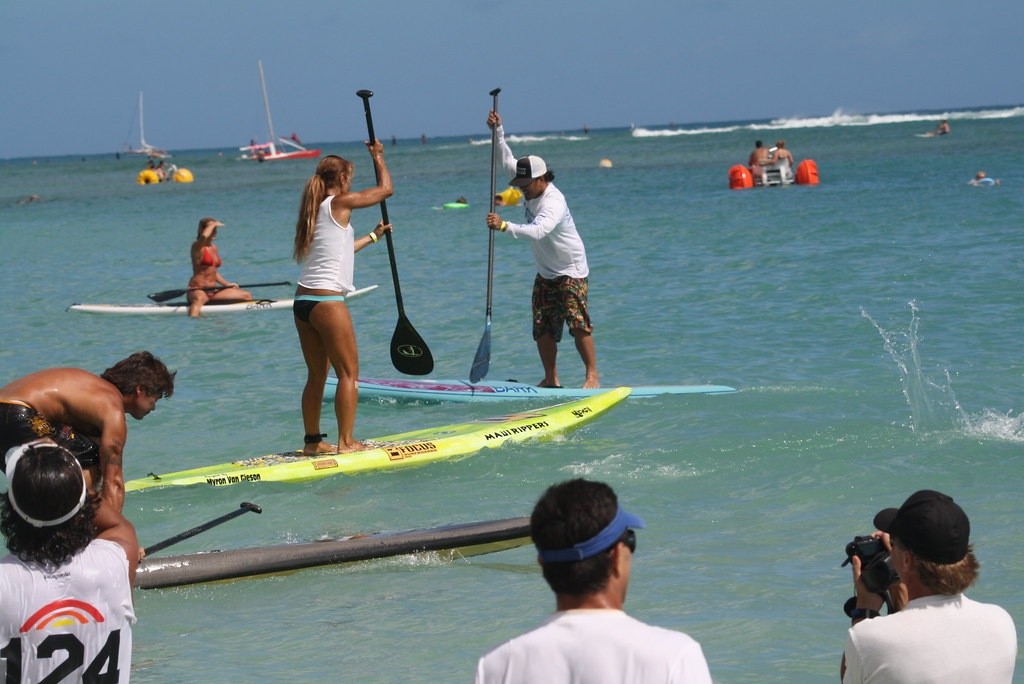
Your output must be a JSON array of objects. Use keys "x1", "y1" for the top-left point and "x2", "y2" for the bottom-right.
[
  {"x1": 236, "y1": 58, "x2": 321, "y2": 163},
  {"x1": 114, "y1": 89, "x2": 174, "y2": 160}
]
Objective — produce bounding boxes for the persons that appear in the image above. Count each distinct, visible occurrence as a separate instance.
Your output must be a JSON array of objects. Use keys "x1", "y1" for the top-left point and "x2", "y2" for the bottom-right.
[
  {"x1": 939, "y1": 121, "x2": 950, "y2": 135},
  {"x1": 186, "y1": 218, "x2": 253, "y2": 317},
  {"x1": 485, "y1": 108, "x2": 602, "y2": 389},
  {"x1": 1, "y1": 433, "x2": 138, "y2": 684},
  {"x1": 148, "y1": 160, "x2": 166, "y2": 180},
  {"x1": 761, "y1": 140, "x2": 793, "y2": 167},
  {"x1": 292, "y1": 139, "x2": 394, "y2": 454},
  {"x1": 0, "y1": 350, "x2": 176, "y2": 564},
  {"x1": 472, "y1": 478, "x2": 713, "y2": 684},
  {"x1": 257, "y1": 148, "x2": 265, "y2": 162},
  {"x1": 970, "y1": 171, "x2": 1001, "y2": 186},
  {"x1": 749, "y1": 140, "x2": 770, "y2": 166},
  {"x1": 841, "y1": 489, "x2": 1017, "y2": 683}
]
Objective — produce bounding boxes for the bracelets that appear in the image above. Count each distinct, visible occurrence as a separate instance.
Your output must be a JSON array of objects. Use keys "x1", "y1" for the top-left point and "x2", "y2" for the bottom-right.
[
  {"x1": 369, "y1": 232, "x2": 378, "y2": 243},
  {"x1": 500, "y1": 221, "x2": 506, "y2": 231}
]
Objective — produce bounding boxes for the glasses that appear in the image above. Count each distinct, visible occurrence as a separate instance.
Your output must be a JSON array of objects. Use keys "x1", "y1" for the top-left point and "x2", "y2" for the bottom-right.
[
  {"x1": 616, "y1": 530, "x2": 636, "y2": 553},
  {"x1": 890, "y1": 533, "x2": 914, "y2": 560}
]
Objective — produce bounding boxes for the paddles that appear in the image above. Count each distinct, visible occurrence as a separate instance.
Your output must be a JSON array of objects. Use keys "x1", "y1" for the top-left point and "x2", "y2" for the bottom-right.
[
  {"x1": 469, "y1": 86, "x2": 502, "y2": 384},
  {"x1": 356, "y1": 90, "x2": 434, "y2": 376},
  {"x1": 144, "y1": 501, "x2": 263, "y2": 558},
  {"x1": 147, "y1": 280, "x2": 292, "y2": 303}
]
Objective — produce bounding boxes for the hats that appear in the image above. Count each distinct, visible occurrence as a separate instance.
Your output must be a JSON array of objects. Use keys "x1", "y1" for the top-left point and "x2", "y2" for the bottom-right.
[
  {"x1": 508, "y1": 155, "x2": 547, "y2": 187},
  {"x1": 538, "y1": 507, "x2": 645, "y2": 562},
  {"x1": 874, "y1": 490, "x2": 970, "y2": 564},
  {"x1": 6, "y1": 441, "x2": 86, "y2": 527}
]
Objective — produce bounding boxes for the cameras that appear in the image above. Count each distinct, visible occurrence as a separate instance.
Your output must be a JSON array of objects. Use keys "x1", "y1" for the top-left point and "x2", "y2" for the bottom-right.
[{"x1": 846, "y1": 534, "x2": 899, "y2": 594}]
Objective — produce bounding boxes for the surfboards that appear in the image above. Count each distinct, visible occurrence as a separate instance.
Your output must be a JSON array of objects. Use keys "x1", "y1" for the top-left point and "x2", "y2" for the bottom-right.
[
  {"x1": 132, "y1": 516, "x2": 534, "y2": 590},
  {"x1": 124, "y1": 386, "x2": 633, "y2": 493},
  {"x1": 321, "y1": 376, "x2": 740, "y2": 404},
  {"x1": 69, "y1": 284, "x2": 379, "y2": 316}
]
